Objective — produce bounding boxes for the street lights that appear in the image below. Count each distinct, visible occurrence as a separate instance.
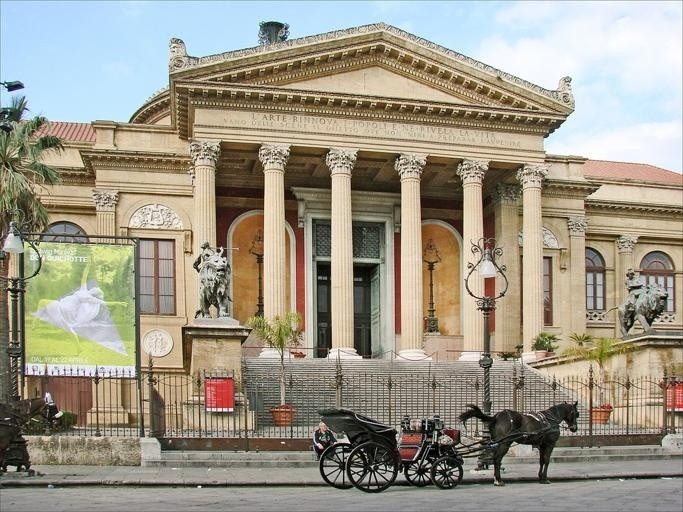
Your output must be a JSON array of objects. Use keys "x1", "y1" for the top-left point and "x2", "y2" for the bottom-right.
[
  {"x1": 463, "y1": 235, "x2": 510, "y2": 472},
  {"x1": 0, "y1": 197, "x2": 32, "y2": 474}
]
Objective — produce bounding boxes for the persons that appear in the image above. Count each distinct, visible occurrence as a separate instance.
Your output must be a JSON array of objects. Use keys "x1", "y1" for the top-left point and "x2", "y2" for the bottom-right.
[
  {"x1": 193, "y1": 241, "x2": 214, "y2": 319},
  {"x1": 313, "y1": 421, "x2": 338, "y2": 461},
  {"x1": 624, "y1": 268, "x2": 647, "y2": 317},
  {"x1": 28, "y1": 257, "x2": 131, "y2": 353}
]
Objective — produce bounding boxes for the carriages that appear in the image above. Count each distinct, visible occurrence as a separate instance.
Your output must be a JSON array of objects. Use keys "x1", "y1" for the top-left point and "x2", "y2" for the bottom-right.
[{"x1": 316, "y1": 401, "x2": 577, "y2": 494}]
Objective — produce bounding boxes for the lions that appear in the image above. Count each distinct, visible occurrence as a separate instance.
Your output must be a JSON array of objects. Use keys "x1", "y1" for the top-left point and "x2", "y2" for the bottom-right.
[
  {"x1": 601, "y1": 282, "x2": 669, "y2": 337},
  {"x1": 193, "y1": 253, "x2": 233, "y2": 319}
]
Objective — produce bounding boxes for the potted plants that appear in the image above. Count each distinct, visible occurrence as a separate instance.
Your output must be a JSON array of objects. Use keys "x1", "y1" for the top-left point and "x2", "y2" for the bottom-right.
[
  {"x1": 589, "y1": 336, "x2": 616, "y2": 422},
  {"x1": 247, "y1": 312, "x2": 306, "y2": 427}
]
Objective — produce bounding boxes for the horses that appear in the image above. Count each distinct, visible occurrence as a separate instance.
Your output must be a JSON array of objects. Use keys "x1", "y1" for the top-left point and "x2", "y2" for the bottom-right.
[
  {"x1": 458, "y1": 400, "x2": 580, "y2": 487},
  {"x1": 0, "y1": 395, "x2": 63, "y2": 469}
]
